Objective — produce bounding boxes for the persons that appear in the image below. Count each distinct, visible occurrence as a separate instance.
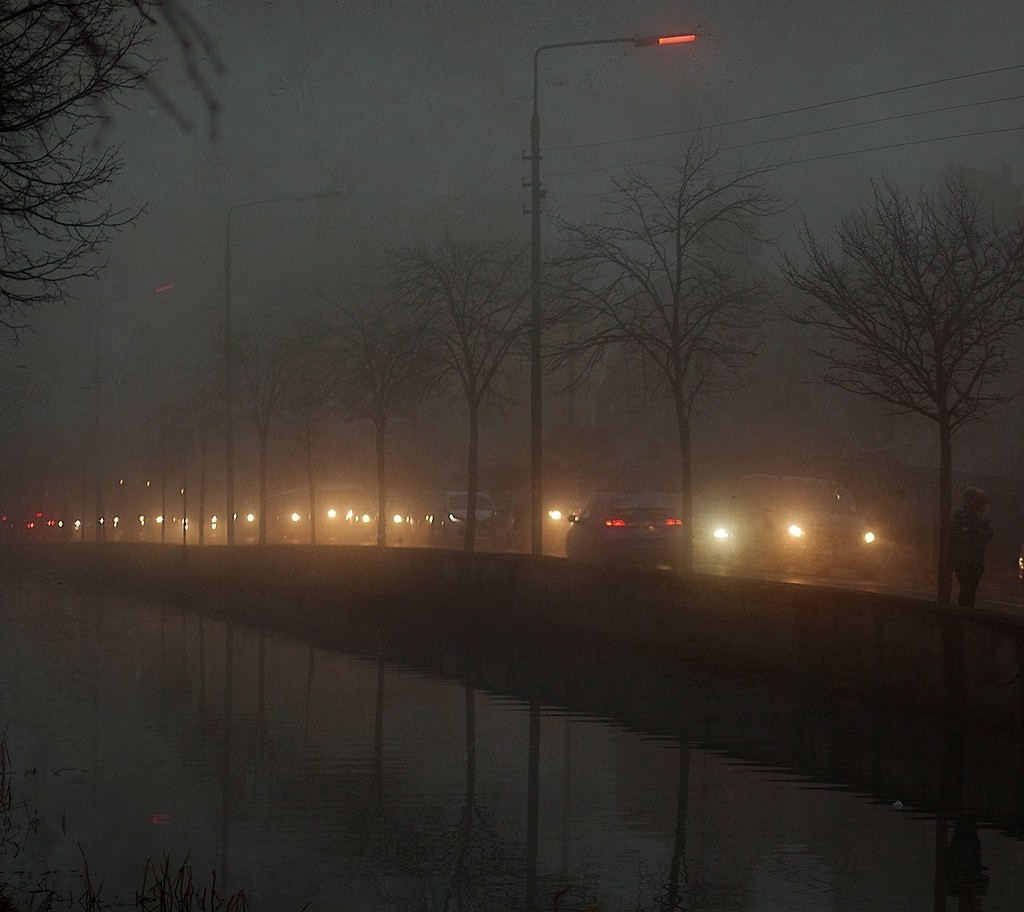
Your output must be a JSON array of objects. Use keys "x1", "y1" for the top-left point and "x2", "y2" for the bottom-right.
[{"x1": 950, "y1": 487, "x2": 993, "y2": 606}]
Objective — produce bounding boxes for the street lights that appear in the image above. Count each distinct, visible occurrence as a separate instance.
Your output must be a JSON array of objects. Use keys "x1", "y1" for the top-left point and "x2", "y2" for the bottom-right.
[
  {"x1": 93, "y1": 281, "x2": 177, "y2": 540},
  {"x1": 224, "y1": 187, "x2": 338, "y2": 539},
  {"x1": 529, "y1": 31, "x2": 698, "y2": 563}
]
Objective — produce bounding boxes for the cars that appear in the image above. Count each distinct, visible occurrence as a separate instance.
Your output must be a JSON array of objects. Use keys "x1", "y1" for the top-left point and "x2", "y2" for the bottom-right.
[
  {"x1": 425, "y1": 485, "x2": 504, "y2": 545},
  {"x1": 565, "y1": 492, "x2": 689, "y2": 566},
  {"x1": 731, "y1": 475, "x2": 884, "y2": 581}
]
